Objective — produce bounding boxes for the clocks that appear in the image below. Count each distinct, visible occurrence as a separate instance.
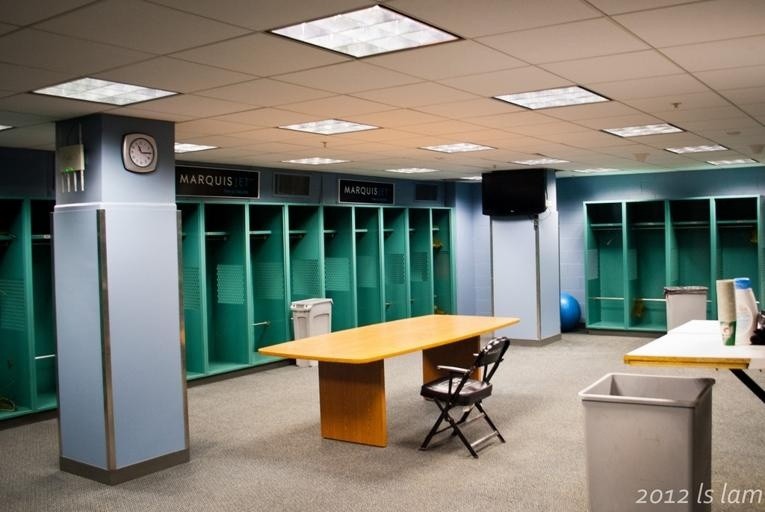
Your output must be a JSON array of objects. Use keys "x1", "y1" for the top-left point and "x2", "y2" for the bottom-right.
[{"x1": 121, "y1": 131, "x2": 159, "y2": 174}]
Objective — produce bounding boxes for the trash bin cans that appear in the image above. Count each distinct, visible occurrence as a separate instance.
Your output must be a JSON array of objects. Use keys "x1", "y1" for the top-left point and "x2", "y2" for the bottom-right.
[
  {"x1": 579, "y1": 372, "x2": 716, "y2": 512},
  {"x1": 663, "y1": 285, "x2": 708, "y2": 334},
  {"x1": 290, "y1": 297, "x2": 334, "y2": 368}
]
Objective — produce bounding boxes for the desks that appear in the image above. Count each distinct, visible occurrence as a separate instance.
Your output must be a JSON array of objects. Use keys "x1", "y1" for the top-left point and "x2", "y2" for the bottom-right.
[
  {"x1": 257, "y1": 314, "x2": 521, "y2": 448},
  {"x1": 623, "y1": 319, "x2": 765, "y2": 404}
]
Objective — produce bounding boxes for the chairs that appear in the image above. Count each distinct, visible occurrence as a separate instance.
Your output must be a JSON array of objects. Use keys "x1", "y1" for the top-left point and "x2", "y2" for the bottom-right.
[{"x1": 420, "y1": 336, "x2": 510, "y2": 459}]
